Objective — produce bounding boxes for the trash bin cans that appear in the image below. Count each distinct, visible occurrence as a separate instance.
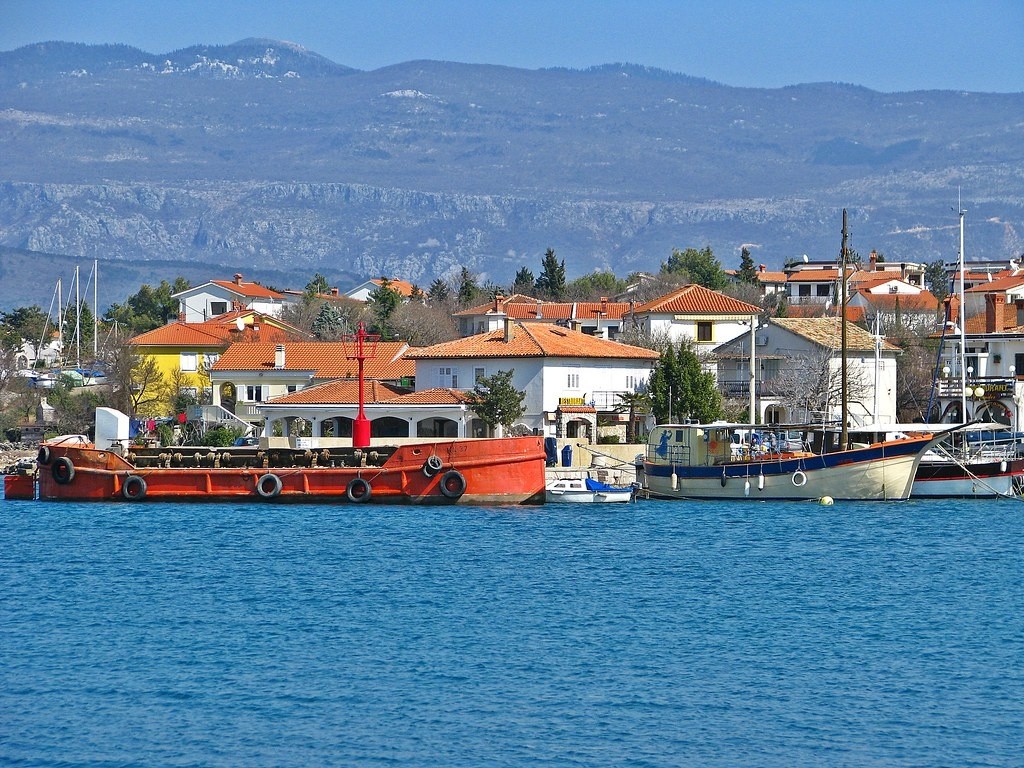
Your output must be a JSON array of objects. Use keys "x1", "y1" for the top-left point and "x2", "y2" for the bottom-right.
[
  {"x1": 561, "y1": 445, "x2": 573, "y2": 467},
  {"x1": 544, "y1": 437, "x2": 558, "y2": 467}
]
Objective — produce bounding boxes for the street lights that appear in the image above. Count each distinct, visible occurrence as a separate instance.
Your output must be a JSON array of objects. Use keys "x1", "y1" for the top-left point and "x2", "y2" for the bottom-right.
[{"x1": 738, "y1": 315, "x2": 768, "y2": 443}]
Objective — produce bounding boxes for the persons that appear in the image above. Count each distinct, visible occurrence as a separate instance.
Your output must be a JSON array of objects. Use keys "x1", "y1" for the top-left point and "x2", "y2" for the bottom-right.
[
  {"x1": 768, "y1": 433, "x2": 777, "y2": 452},
  {"x1": 750, "y1": 439, "x2": 758, "y2": 457}
]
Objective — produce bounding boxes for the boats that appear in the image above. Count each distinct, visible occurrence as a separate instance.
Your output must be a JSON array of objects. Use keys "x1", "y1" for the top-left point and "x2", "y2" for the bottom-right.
[
  {"x1": 34, "y1": 436, "x2": 546, "y2": 505},
  {"x1": 547, "y1": 477, "x2": 642, "y2": 504}
]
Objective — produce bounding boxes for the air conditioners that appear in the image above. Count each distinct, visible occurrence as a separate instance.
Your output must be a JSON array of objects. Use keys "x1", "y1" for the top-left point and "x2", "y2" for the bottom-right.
[{"x1": 755, "y1": 336, "x2": 767, "y2": 345}]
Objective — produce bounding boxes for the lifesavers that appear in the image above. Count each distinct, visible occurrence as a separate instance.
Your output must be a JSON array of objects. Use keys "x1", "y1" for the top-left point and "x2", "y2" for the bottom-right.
[
  {"x1": 38, "y1": 446, "x2": 51, "y2": 465},
  {"x1": 257, "y1": 473, "x2": 283, "y2": 499},
  {"x1": 346, "y1": 478, "x2": 372, "y2": 503},
  {"x1": 52, "y1": 456, "x2": 75, "y2": 485},
  {"x1": 422, "y1": 455, "x2": 443, "y2": 478},
  {"x1": 438, "y1": 470, "x2": 467, "y2": 499},
  {"x1": 122, "y1": 475, "x2": 147, "y2": 501},
  {"x1": 791, "y1": 471, "x2": 807, "y2": 487}
]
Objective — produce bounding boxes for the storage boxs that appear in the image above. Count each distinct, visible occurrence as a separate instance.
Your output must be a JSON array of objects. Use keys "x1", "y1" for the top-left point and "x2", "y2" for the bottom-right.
[{"x1": 296, "y1": 438, "x2": 308, "y2": 448}]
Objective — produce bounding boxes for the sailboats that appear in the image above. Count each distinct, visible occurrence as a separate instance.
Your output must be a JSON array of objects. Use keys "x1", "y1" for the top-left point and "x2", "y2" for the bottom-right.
[
  {"x1": 1, "y1": 259, "x2": 123, "y2": 390},
  {"x1": 636, "y1": 207, "x2": 1024, "y2": 502}
]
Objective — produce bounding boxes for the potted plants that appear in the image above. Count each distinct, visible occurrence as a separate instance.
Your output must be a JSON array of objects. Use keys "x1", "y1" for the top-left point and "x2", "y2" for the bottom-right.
[
  {"x1": 132, "y1": 438, "x2": 145, "y2": 448},
  {"x1": 993, "y1": 355, "x2": 1000, "y2": 363}
]
